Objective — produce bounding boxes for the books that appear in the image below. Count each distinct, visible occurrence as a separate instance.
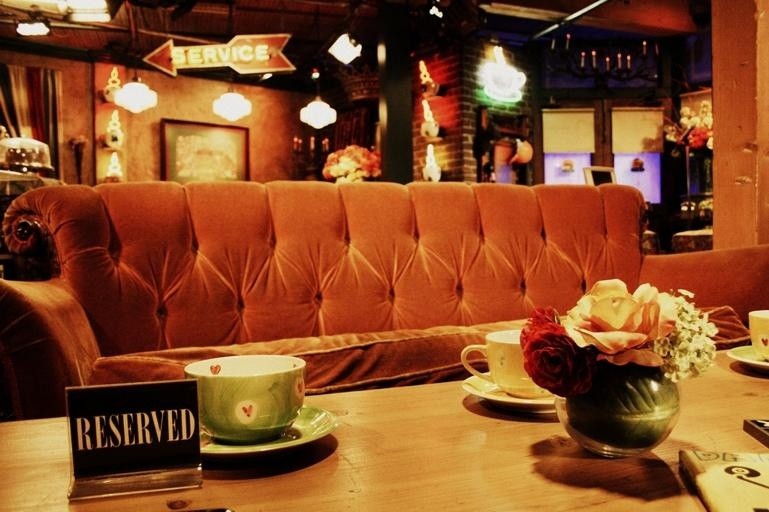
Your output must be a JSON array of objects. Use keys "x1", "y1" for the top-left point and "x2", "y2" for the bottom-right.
[
  {"x1": 675, "y1": 443, "x2": 769, "y2": 512},
  {"x1": 742, "y1": 413, "x2": 768, "y2": 449}
]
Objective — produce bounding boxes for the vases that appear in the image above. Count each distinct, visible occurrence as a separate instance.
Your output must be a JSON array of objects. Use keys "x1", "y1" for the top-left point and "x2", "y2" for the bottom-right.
[
  {"x1": 686, "y1": 147, "x2": 706, "y2": 201},
  {"x1": 555, "y1": 365, "x2": 680, "y2": 460}
]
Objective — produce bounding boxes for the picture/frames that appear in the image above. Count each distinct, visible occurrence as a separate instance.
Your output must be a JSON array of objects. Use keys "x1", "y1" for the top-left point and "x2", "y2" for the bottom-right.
[{"x1": 161, "y1": 118, "x2": 250, "y2": 182}]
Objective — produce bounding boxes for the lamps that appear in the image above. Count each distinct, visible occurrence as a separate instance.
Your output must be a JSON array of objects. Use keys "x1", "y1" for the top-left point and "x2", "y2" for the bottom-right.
[
  {"x1": 212, "y1": 80, "x2": 253, "y2": 123},
  {"x1": 415, "y1": 61, "x2": 449, "y2": 181},
  {"x1": 328, "y1": 26, "x2": 363, "y2": 66},
  {"x1": 16, "y1": 4, "x2": 51, "y2": 37},
  {"x1": 298, "y1": 83, "x2": 338, "y2": 130},
  {"x1": 114, "y1": 61, "x2": 158, "y2": 115},
  {"x1": 98, "y1": 66, "x2": 126, "y2": 184}
]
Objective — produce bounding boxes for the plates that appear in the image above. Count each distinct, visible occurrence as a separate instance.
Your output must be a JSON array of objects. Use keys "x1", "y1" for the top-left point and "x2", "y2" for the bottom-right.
[
  {"x1": 461, "y1": 371, "x2": 556, "y2": 414},
  {"x1": 195, "y1": 403, "x2": 338, "y2": 456},
  {"x1": 727, "y1": 345, "x2": 769, "y2": 372}
]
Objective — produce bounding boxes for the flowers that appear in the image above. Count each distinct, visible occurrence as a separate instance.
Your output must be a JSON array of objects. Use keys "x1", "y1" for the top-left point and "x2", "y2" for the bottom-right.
[
  {"x1": 521, "y1": 279, "x2": 718, "y2": 400},
  {"x1": 663, "y1": 86, "x2": 713, "y2": 156},
  {"x1": 322, "y1": 145, "x2": 381, "y2": 181}
]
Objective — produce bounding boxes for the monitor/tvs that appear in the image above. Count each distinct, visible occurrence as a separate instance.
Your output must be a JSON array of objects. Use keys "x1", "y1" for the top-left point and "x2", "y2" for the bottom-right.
[{"x1": 583, "y1": 166, "x2": 617, "y2": 187}]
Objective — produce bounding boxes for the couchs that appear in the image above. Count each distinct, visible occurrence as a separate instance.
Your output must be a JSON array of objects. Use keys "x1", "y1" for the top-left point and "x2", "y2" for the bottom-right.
[{"x1": 0, "y1": 181, "x2": 767, "y2": 406}]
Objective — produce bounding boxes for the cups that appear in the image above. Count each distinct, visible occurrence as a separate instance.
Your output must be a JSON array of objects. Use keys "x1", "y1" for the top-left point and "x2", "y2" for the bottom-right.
[
  {"x1": 461, "y1": 329, "x2": 556, "y2": 397},
  {"x1": 749, "y1": 310, "x2": 769, "y2": 362},
  {"x1": 186, "y1": 354, "x2": 306, "y2": 445}
]
